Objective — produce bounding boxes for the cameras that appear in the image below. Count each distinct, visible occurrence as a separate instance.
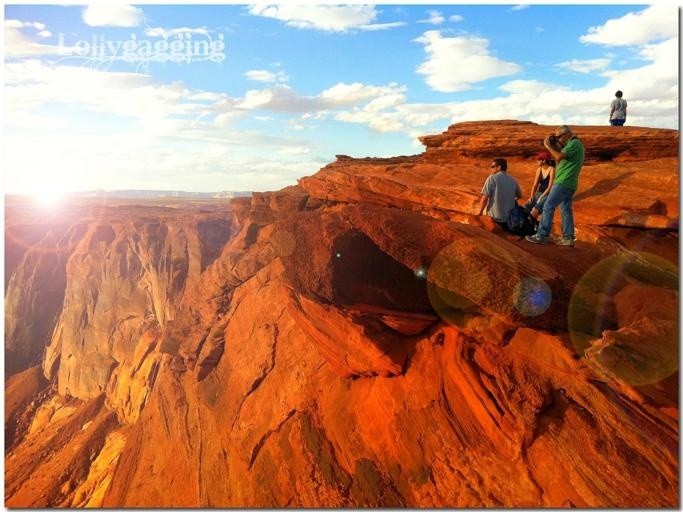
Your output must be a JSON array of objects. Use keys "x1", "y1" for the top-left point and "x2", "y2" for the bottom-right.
[{"x1": 548, "y1": 136, "x2": 557, "y2": 145}]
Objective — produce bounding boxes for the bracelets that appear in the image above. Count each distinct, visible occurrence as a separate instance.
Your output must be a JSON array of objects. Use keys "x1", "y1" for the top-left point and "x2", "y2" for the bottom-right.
[{"x1": 609, "y1": 113, "x2": 612, "y2": 117}]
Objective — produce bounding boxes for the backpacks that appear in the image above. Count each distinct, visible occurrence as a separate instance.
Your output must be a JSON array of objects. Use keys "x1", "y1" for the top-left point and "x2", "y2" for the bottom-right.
[{"x1": 506, "y1": 200, "x2": 540, "y2": 237}]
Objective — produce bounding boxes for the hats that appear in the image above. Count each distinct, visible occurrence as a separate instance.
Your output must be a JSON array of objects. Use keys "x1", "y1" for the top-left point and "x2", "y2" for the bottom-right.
[{"x1": 535, "y1": 151, "x2": 551, "y2": 163}]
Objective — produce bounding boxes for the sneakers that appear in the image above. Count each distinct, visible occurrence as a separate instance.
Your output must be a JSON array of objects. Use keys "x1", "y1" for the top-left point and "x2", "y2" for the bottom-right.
[
  {"x1": 525, "y1": 232, "x2": 546, "y2": 244},
  {"x1": 555, "y1": 236, "x2": 575, "y2": 246}
]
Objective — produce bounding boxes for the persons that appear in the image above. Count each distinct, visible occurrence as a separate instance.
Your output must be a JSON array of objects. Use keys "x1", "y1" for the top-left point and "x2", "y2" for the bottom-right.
[
  {"x1": 608, "y1": 90, "x2": 627, "y2": 128},
  {"x1": 523, "y1": 152, "x2": 556, "y2": 219},
  {"x1": 523, "y1": 124, "x2": 585, "y2": 248},
  {"x1": 474, "y1": 158, "x2": 523, "y2": 232}
]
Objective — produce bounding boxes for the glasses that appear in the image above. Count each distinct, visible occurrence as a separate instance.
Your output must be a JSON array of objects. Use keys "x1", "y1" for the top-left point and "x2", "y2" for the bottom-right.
[
  {"x1": 490, "y1": 164, "x2": 496, "y2": 169},
  {"x1": 553, "y1": 132, "x2": 564, "y2": 139}
]
